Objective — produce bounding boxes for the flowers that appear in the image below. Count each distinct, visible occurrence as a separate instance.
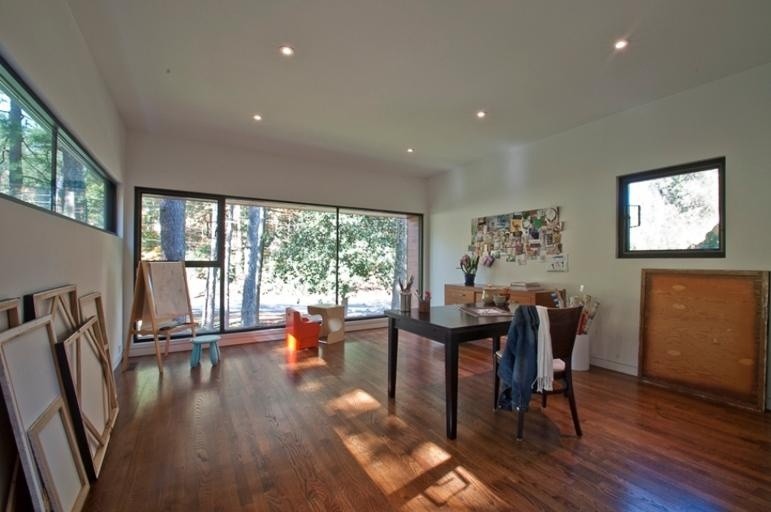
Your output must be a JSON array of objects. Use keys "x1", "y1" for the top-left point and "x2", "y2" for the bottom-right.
[
  {"x1": 413, "y1": 287, "x2": 433, "y2": 303},
  {"x1": 456, "y1": 254, "x2": 482, "y2": 275},
  {"x1": 555, "y1": 287, "x2": 602, "y2": 334}
]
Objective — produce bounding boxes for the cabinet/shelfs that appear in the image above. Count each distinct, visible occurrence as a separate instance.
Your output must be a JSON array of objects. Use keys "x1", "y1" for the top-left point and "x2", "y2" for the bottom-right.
[{"x1": 444, "y1": 282, "x2": 559, "y2": 352}]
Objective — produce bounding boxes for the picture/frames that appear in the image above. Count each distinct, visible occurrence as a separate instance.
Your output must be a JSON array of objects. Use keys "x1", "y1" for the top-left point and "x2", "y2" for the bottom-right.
[{"x1": 0, "y1": 283, "x2": 121, "y2": 512}]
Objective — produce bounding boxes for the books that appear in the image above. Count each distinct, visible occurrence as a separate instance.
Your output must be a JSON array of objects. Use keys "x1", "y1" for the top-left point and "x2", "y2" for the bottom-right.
[{"x1": 509, "y1": 280, "x2": 546, "y2": 292}]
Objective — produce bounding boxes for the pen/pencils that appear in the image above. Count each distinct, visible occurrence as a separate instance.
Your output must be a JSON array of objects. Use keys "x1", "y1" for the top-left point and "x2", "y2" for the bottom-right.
[
  {"x1": 414, "y1": 289, "x2": 431, "y2": 303},
  {"x1": 398, "y1": 273, "x2": 414, "y2": 293}
]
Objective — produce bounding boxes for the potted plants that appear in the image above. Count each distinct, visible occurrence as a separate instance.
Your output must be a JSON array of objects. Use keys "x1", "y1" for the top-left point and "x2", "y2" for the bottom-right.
[{"x1": 396, "y1": 272, "x2": 415, "y2": 313}]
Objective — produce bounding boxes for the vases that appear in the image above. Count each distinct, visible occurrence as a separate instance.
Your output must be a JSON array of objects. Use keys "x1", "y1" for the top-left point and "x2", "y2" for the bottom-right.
[
  {"x1": 419, "y1": 300, "x2": 431, "y2": 314},
  {"x1": 464, "y1": 273, "x2": 476, "y2": 286}
]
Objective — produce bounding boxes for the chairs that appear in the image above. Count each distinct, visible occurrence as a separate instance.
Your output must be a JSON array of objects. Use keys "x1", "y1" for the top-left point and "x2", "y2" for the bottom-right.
[
  {"x1": 285, "y1": 306, "x2": 322, "y2": 353},
  {"x1": 493, "y1": 304, "x2": 584, "y2": 442}
]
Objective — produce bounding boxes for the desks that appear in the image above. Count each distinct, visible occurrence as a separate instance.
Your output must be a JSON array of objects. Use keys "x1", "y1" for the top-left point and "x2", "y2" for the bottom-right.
[
  {"x1": 307, "y1": 303, "x2": 348, "y2": 345},
  {"x1": 384, "y1": 303, "x2": 514, "y2": 441}
]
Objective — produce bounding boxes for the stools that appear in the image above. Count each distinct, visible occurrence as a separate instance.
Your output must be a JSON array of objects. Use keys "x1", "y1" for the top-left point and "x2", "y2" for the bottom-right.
[{"x1": 189, "y1": 333, "x2": 223, "y2": 369}]
